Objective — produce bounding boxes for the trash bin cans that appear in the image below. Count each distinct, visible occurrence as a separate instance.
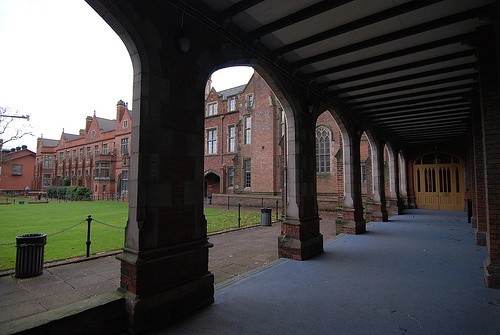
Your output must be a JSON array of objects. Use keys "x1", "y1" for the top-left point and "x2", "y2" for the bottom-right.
[
  {"x1": 468, "y1": 198, "x2": 472, "y2": 224},
  {"x1": 15, "y1": 233, "x2": 47, "y2": 278},
  {"x1": 260, "y1": 208, "x2": 272, "y2": 226}
]
